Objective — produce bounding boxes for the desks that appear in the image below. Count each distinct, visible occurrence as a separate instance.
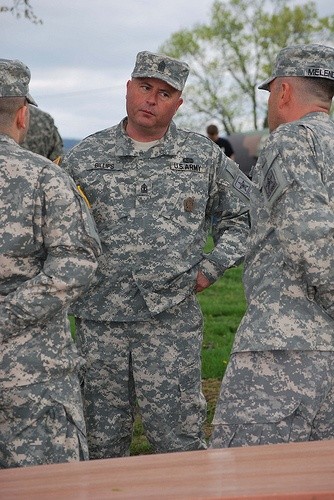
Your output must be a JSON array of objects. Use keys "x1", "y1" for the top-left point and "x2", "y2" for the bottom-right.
[{"x1": 0, "y1": 439, "x2": 334, "y2": 500}]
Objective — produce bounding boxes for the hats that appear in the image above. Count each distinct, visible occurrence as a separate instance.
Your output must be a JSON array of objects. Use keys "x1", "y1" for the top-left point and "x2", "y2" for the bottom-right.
[
  {"x1": 258, "y1": 44, "x2": 334, "y2": 91},
  {"x1": 0, "y1": 59, "x2": 38, "y2": 108},
  {"x1": 131, "y1": 51, "x2": 190, "y2": 91}
]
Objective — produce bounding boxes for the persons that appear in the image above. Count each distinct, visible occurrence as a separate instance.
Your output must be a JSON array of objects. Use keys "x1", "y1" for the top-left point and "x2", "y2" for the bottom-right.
[
  {"x1": 206, "y1": 125, "x2": 234, "y2": 161},
  {"x1": 51, "y1": 51, "x2": 251, "y2": 461},
  {"x1": 0, "y1": 58, "x2": 102, "y2": 469},
  {"x1": 208, "y1": 43, "x2": 334, "y2": 449}
]
